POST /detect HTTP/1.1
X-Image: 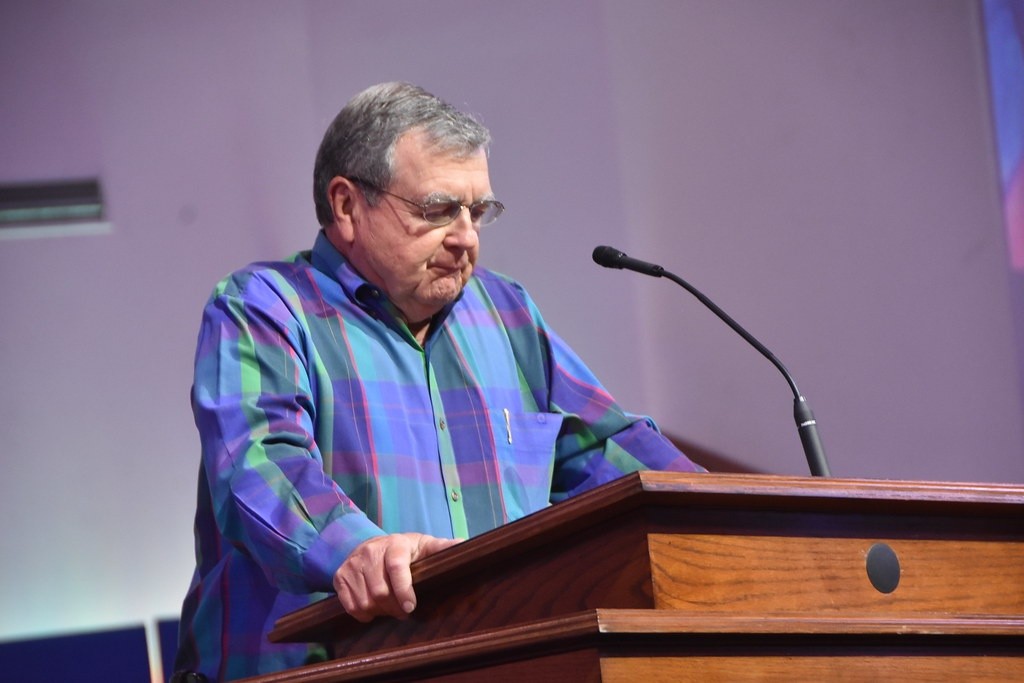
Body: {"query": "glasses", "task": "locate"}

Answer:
[347,176,506,227]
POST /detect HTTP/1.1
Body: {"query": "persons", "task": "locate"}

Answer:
[173,81,708,683]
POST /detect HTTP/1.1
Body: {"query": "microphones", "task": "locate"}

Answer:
[592,245,830,479]
